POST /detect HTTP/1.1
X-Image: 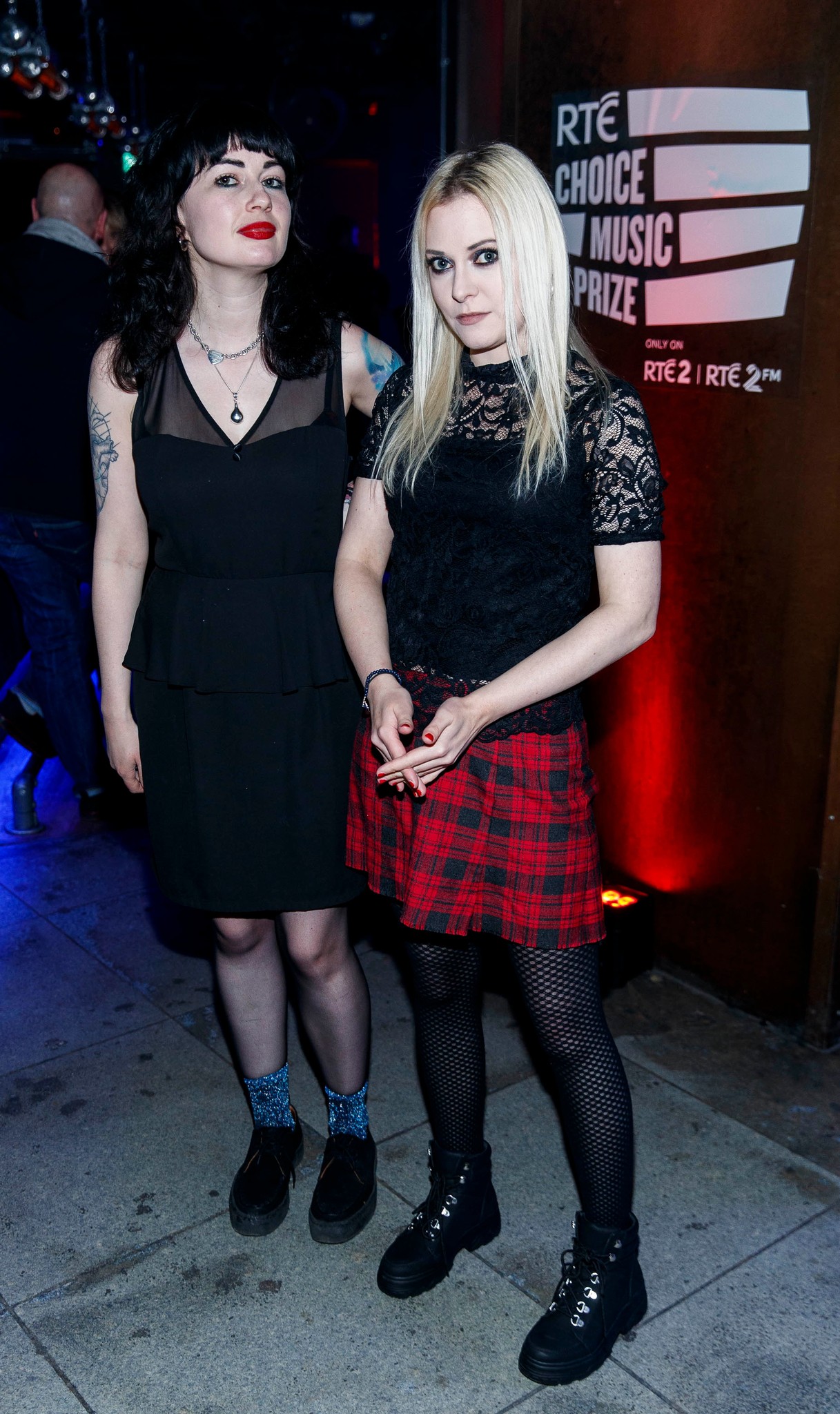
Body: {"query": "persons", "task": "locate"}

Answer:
[89,100,405,1245]
[333,146,663,1385]
[0,156,132,848]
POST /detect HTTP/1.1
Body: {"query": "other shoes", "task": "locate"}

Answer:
[80,788,149,829]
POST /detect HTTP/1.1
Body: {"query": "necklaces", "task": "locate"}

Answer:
[183,315,265,364]
[185,311,264,425]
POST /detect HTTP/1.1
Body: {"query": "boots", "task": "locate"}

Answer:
[518,1210,645,1386]
[379,1142,502,1299]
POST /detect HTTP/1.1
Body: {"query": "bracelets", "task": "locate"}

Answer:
[361,668,402,713]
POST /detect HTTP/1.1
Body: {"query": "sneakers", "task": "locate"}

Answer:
[308,1124,376,1246]
[228,1106,302,1236]
